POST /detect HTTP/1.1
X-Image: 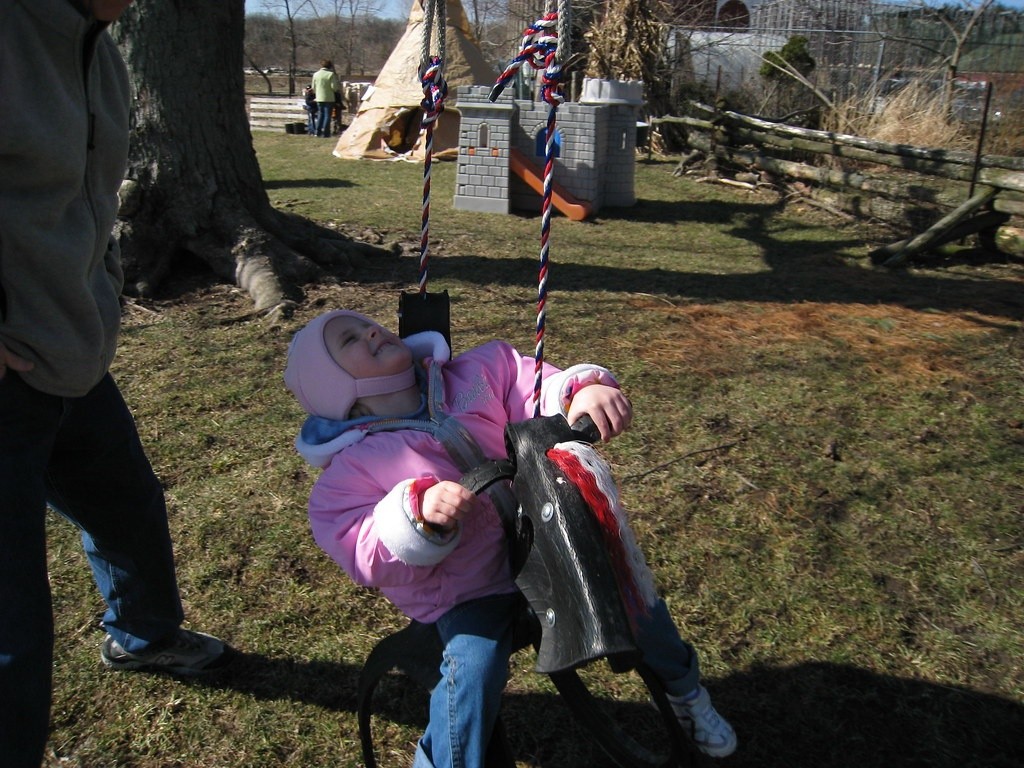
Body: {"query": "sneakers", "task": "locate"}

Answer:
[100,627,235,675]
[649,682,737,758]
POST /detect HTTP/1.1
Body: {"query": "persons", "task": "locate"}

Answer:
[304,61,346,139]
[285,312,738,768]
[0,1,239,768]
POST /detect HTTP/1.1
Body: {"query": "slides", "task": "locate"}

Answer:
[511,148,593,223]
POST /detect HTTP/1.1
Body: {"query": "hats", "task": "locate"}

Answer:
[282,310,415,420]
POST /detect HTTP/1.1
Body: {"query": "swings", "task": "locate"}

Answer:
[355,0,684,768]
